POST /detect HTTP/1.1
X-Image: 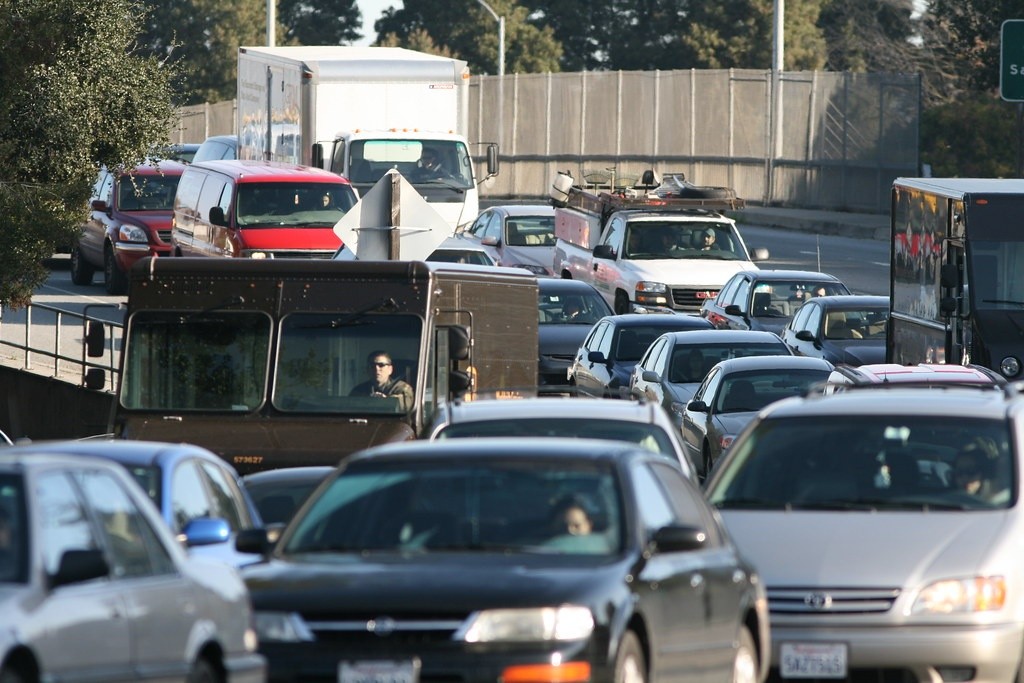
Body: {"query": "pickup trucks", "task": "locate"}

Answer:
[551,206,771,315]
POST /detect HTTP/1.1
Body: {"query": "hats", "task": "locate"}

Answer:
[704,228,716,237]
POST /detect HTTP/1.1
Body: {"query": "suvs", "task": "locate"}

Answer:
[70,157,187,296]
[822,362,1024,500]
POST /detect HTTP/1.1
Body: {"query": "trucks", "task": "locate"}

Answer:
[235,44,499,239]
[886,179,1023,383]
[85,254,539,486]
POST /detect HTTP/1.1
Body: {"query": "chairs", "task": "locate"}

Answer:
[391,360,418,389]
[363,477,463,548]
[447,476,503,548]
[348,158,372,183]
[753,293,784,316]
[562,298,586,319]
[169,351,258,410]
[828,320,854,338]
[724,381,757,409]
[508,222,527,245]
[619,332,639,359]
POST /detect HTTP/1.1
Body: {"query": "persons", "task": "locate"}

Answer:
[549,494,593,538]
[409,147,452,183]
[948,451,991,509]
[678,348,706,381]
[556,296,586,324]
[810,286,826,298]
[350,349,413,425]
[313,193,337,212]
[629,227,721,255]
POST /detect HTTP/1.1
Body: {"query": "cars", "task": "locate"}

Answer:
[429,396,704,496]
[0,437,337,587]
[536,278,622,381]
[700,395,1023,682]
[785,295,890,367]
[151,135,239,167]
[467,205,561,277]
[570,313,719,400]
[629,328,800,430]
[0,456,264,683]
[680,356,836,482]
[239,439,770,683]
[699,269,857,342]
[425,241,497,268]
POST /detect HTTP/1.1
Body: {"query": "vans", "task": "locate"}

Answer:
[172,159,361,262]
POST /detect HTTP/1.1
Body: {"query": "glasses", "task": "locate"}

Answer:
[422,156,433,160]
[369,363,391,367]
